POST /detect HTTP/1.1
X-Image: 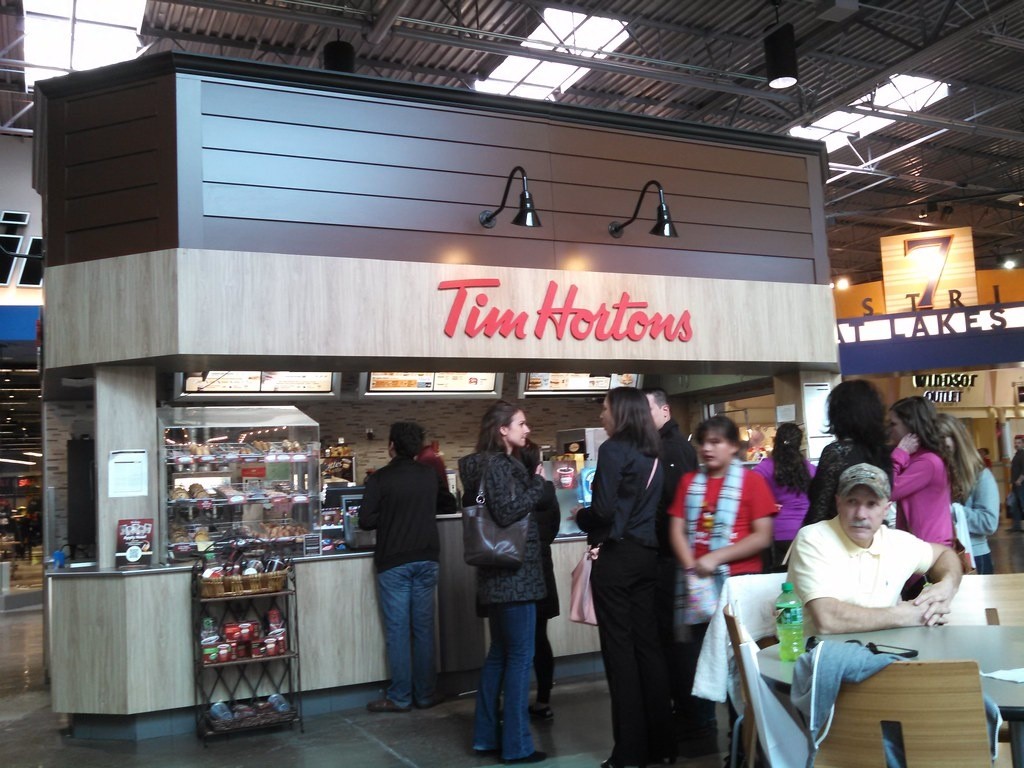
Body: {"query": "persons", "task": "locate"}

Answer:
[459,400,560,764]
[640,387,719,759]
[938,414,1001,574]
[801,380,894,528]
[887,395,953,547]
[358,423,456,712]
[418,437,449,491]
[667,416,779,766]
[567,387,680,767]
[785,463,962,637]
[1006,435,1024,533]
[752,424,816,573]
[978,448,991,469]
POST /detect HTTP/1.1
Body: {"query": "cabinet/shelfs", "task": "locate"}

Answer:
[157,406,322,564]
[190,535,304,748]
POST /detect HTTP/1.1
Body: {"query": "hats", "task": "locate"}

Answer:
[1015,435,1024,439]
[837,463,891,503]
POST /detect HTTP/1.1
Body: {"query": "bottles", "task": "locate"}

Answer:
[774,582,806,662]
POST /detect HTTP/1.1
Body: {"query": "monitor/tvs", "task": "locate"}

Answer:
[341,494,365,543]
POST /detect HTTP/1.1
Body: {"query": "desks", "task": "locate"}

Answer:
[756,625,1024,768]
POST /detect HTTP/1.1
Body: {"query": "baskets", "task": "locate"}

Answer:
[206,705,297,731]
[198,566,292,598]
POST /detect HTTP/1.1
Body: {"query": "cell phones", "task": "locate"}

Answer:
[874,644,919,658]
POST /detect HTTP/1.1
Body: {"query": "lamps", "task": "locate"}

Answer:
[324,29,354,73]
[479,166,542,229]
[763,0,799,89]
[608,180,677,238]
[941,206,953,223]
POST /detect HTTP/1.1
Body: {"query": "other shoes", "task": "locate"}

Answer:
[503,751,546,764]
[413,696,437,709]
[368,699,411,711]
[474,744,502,756]
[528,704,554,721]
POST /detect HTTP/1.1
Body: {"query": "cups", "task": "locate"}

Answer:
[201,557,288,664]
[556,467,575,488]
[210,702,232,720]
[267,693,289,711]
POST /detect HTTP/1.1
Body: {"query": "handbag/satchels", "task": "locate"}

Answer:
[568,548,599,626]
[949,503,977,575]
[463,452,531,571]
[683,563,730,626]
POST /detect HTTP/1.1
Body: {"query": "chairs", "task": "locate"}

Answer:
[691,572,1023,767]
